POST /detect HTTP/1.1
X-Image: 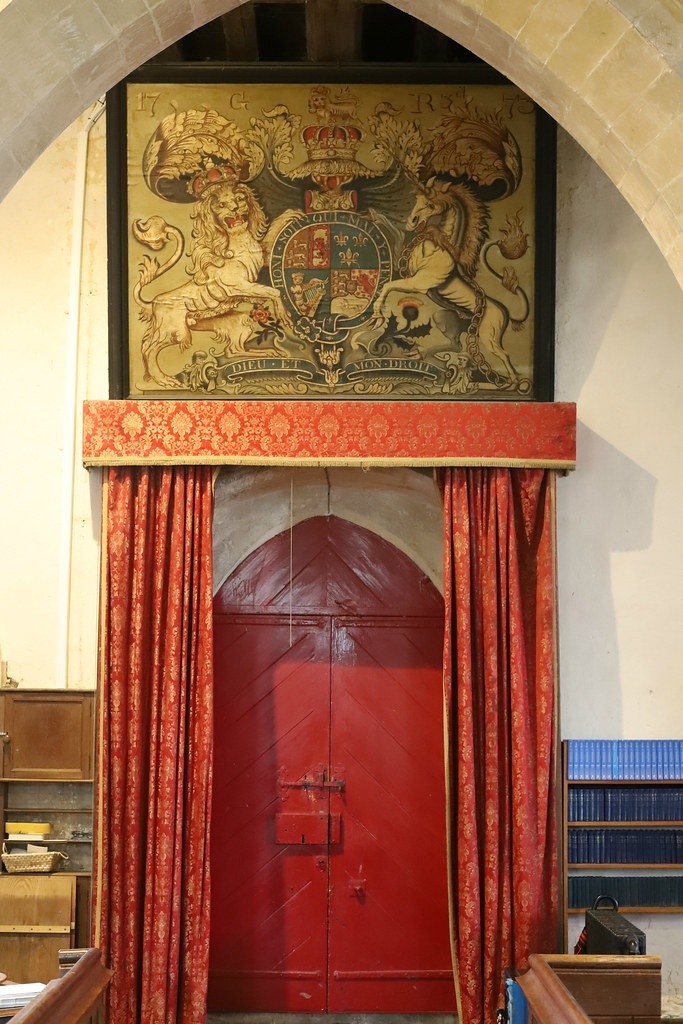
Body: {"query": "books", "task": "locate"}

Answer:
[568,740,683,908]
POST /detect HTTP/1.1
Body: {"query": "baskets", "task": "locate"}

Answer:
[1,843,68,873]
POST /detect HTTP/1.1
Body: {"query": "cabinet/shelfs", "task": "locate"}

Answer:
[0,689,97,947]
[563,739,683,954]
